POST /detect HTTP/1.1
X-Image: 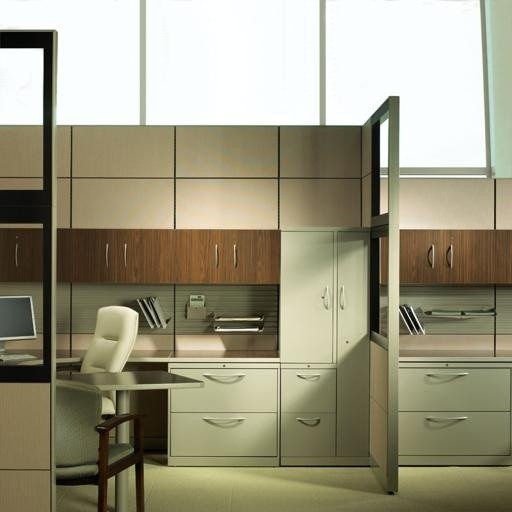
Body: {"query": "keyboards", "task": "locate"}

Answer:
[0,354,39,362]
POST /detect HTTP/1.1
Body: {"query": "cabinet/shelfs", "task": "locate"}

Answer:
[398,357,512,466]
[68,229,173,284]
[173,229,280,285]
[168,362,280,466]
[379,229,512,285]
[280,229,370,467]
[0,227,68,283]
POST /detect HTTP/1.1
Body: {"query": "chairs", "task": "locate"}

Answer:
[80,305,139,416]
[56,378,145,512]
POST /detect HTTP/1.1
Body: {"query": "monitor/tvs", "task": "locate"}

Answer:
[0,296,37,353]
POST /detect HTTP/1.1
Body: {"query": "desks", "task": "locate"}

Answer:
[57,371,204,512]
